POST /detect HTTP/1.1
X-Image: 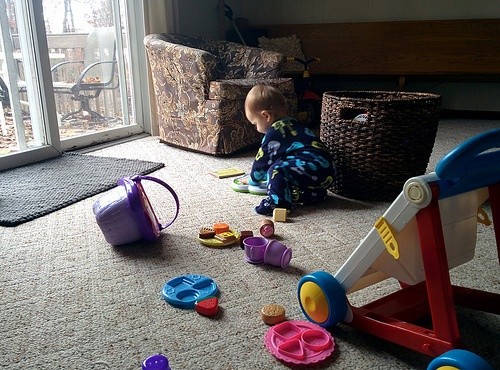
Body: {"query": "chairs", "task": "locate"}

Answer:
[50,29,123,124]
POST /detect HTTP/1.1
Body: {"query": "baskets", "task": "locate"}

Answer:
[319,88,442,201]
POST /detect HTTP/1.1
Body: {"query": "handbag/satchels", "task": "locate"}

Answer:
[93,174,180,246]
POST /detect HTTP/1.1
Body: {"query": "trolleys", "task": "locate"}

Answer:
[297,128,500,370]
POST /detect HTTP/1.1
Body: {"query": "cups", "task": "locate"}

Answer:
[242,236,268,264]
[263,239,292,269]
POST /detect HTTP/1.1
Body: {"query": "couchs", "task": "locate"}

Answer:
[143,33,295,156]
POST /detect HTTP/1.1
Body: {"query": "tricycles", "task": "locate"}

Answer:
[222,2,323,130]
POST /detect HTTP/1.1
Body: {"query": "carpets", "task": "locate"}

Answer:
[0,153,165,226]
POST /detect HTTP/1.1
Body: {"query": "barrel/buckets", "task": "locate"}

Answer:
[92,175,179,248]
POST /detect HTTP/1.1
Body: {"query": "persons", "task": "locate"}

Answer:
[241,84,337,215]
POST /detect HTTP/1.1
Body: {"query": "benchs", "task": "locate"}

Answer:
[242,18,499,92]
